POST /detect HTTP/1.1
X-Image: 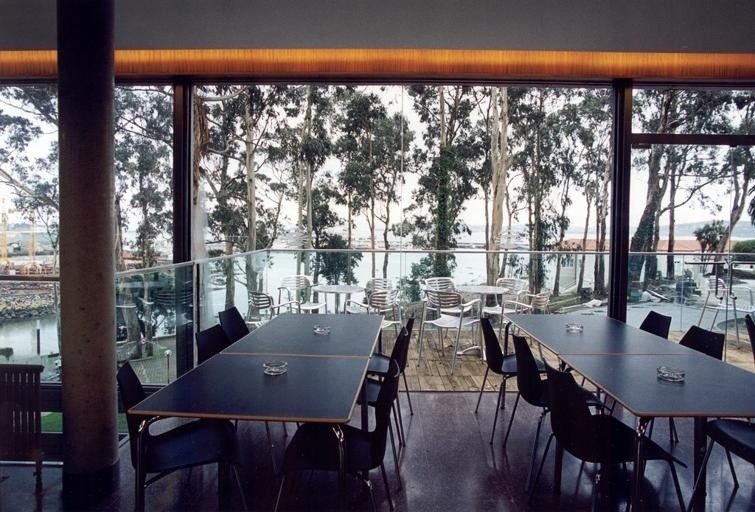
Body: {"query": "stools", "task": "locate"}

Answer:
[686,419,755,512]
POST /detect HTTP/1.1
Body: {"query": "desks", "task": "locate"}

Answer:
[129,353,371,512]
[219,313,387,482]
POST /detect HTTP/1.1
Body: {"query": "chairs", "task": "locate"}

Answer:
[273,358,401,512]
[745,315,755,358]
[116,361,249,511]
[356,326,410,490]
[276,275,327,314]
[367,312,417,416]
[0,364,44,495]
[219,307,250,343]
[697,277,755,350]
[195,324,288,447]
[362,278,399,318]
[246,292,301,323]
[646,326,725,449]
[526,359,686,512]
[602,311,672,416]
[344,290,399,338]
